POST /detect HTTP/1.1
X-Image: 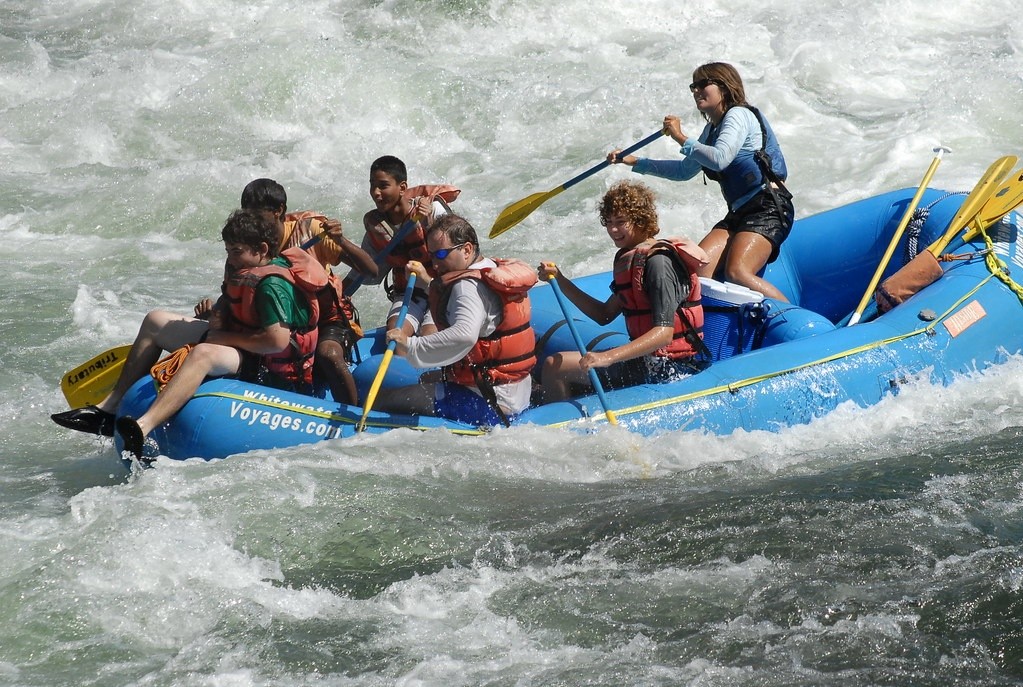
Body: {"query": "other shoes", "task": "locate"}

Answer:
[116,415,144,462]
[51,404,117,438]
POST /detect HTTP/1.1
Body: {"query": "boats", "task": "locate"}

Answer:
[113,187,1023,461]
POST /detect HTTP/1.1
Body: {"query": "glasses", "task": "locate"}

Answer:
[427,244,465,260]
[689,78,725,93]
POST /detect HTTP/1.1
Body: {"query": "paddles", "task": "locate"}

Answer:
[340,212,424,299]
[59,228,334,413]
[545,262,620,427]
[488,125,672,240]
[354,259,419,435]
[834,143,1023,329]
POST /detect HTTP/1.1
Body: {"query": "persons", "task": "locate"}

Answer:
[536,178,711,405]
[372,214,540,425]
[194,178,379,406]
[50,208,328,461]
[341,155,462,357]
[606,62,795,304]
